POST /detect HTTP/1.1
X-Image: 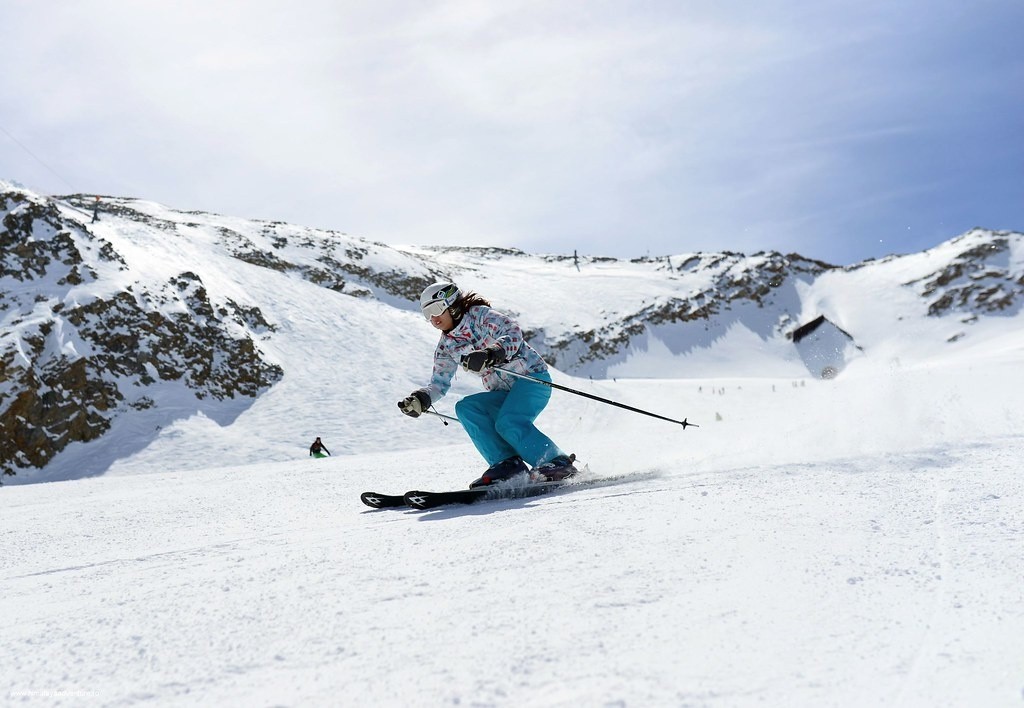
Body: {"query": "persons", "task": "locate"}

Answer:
[309,437,331,458]
[397,282,581,491]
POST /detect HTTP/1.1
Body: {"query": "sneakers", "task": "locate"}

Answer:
[529,455,578,483]
[469,456,529,490]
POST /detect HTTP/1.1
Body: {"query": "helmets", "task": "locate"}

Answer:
[420,282,462,322]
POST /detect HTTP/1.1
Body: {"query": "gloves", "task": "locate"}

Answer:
[462,344,506,375]
[397,390,432,417]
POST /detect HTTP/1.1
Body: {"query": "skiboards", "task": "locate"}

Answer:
[360,466,667,510]
